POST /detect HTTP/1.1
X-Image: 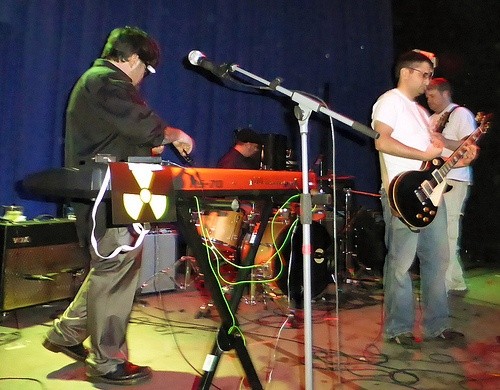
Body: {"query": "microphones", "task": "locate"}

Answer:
[188,50,232,81]
[23,273,54,281]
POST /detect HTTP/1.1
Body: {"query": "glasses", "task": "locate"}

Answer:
[138,56,156,77]
[406,66,433,80]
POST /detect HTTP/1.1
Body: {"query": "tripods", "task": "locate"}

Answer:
[328,186,381,287]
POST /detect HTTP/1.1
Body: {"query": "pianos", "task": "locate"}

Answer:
[164,161,318,193]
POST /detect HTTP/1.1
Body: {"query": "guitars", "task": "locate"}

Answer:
[388,111,494,231]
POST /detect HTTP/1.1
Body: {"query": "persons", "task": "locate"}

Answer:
[205,128,264,226]
[371,50,477,349]
[40,26,193,386]
[417,78,478,301]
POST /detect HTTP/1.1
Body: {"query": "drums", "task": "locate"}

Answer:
[254,217,336,308]
[193,203,251,253]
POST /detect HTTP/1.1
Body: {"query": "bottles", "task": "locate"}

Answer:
[260,144,267,170]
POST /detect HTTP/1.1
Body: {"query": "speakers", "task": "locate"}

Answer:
[0,218,91,312]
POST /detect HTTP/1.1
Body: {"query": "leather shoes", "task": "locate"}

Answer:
[86,359,153,386]
[41,334,89,362]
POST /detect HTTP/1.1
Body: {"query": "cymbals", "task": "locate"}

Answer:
[317,170,356,184]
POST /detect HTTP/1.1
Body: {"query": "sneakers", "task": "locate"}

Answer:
[424,329,465,344]
[384,332,425,349]
